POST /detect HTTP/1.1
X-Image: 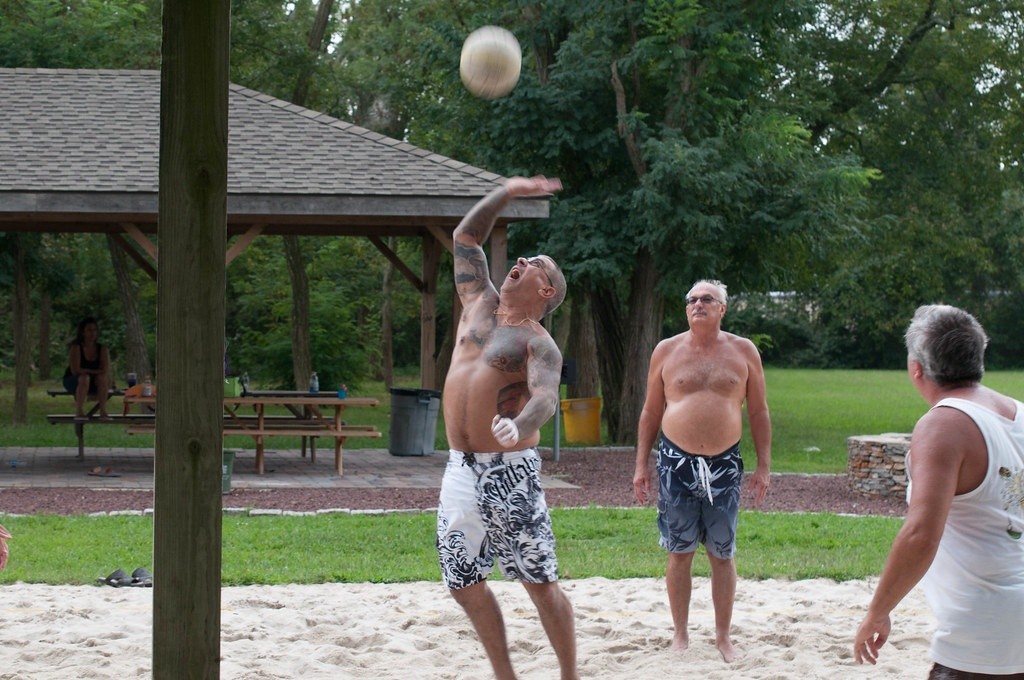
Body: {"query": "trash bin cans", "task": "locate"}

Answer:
[223,376,242,398]
[389,386,442,456]
[560,397,601,447]
[221,450,237,494]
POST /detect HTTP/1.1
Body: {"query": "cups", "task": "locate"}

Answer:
[338,387,346,398]
[129,373,136,387]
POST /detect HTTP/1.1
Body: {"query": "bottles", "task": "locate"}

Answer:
[309,372,319,392]
[142,376,151,397]
[243,372,249,390]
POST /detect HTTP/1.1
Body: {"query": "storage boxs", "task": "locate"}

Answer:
[223,375,242,398]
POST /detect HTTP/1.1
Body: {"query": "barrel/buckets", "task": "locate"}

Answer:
[559,396,601,445]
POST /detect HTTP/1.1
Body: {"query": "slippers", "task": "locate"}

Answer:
[132,568,152,587]
[98,568,145,587]
[98,467,121,476]
[88,466,101,475]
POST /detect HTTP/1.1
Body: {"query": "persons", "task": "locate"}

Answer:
[852,302,1024,680]
[435,176,582,680]
[633,281,773,662]
[62,316,114,423]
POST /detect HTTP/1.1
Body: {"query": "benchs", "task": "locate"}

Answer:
[46,414,383,448]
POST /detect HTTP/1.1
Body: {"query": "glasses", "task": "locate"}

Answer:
[686,295,722,305]
[528,259,553,287]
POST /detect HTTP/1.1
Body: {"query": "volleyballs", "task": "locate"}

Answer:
[459,25,523,102]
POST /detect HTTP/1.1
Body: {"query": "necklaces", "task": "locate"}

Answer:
[494,307,542,326]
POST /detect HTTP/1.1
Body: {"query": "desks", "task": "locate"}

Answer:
[47,387,127,461]
[242,390,345,463]
[125,395,378,474]
